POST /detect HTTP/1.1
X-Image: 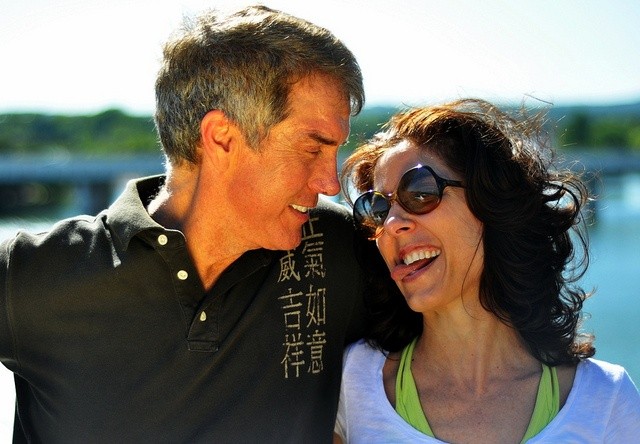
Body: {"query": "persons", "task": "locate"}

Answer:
[0,6,423,444]
[333,98,640,444]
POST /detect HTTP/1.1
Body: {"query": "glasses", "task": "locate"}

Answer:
[353,165,467,230]
